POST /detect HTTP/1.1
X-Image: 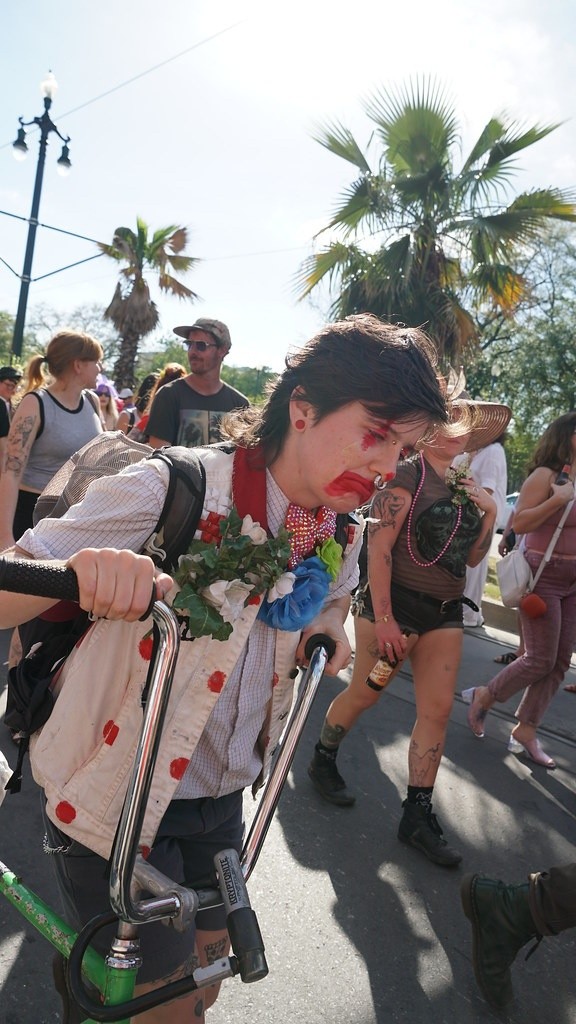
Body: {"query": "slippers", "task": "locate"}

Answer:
[494,651,518,664]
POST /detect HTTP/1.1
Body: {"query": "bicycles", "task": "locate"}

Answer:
[0,554,339,1024]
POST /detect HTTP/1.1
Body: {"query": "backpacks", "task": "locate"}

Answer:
[7,430,347,735]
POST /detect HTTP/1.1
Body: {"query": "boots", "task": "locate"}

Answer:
[308,743,356,805]
[463,869,542,1011]
[398,798,462,865]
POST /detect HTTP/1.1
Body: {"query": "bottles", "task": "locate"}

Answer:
[364,627,412,691]
[549,463,571,498]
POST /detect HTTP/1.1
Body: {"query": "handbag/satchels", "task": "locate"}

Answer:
[496,552,535,606]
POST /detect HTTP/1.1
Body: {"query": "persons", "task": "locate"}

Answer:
[0,313,479,1024]
[0,331,105,672]
[450,451,479,471]
[461,413,576,769]
[307,390,512,866]
[0,364,24,439]
[142,317,251,449]
[125,363,188,443]
[564,683,576,693]
[493,467,527,663]
[460,862,576,1024]
[113,371,165,436]
[118,388,135,408]
[92,381,119,431]
[462,429,511,627]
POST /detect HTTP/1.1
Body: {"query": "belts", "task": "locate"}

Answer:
[389,581,481,614]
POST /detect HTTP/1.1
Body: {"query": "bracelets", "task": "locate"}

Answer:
[375,614,393,622]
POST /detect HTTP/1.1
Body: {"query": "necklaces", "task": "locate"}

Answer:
[406,451,462,567]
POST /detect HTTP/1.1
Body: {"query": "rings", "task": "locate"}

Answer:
[385,643,391,649]
[473,485,478,492]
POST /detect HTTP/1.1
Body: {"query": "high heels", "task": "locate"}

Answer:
[507,734,556,767]
[463,688,488,737]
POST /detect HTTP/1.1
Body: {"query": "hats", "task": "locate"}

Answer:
[173,318,232,351]
[441,385,514,453]
[0,367,26,379]
[119,387,134,399]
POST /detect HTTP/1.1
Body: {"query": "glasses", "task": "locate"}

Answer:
[182,340,218,352]
[95,392,111,398]
[3,381,18,389]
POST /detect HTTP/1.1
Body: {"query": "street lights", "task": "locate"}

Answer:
[6,66,74,368]
